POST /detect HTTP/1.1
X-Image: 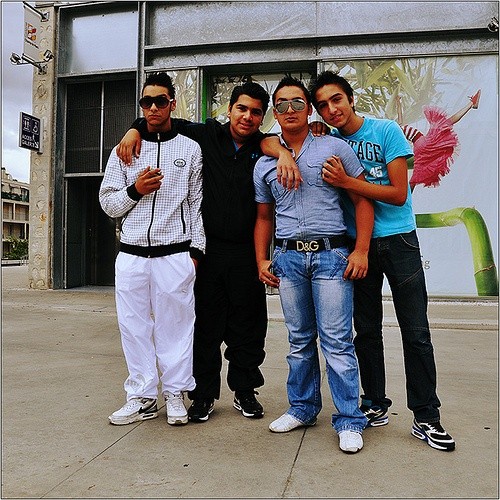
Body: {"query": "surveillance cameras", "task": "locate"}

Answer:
[9,51,21,64]
[42,49,55,61]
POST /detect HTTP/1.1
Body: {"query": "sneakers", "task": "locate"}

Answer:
[233,394,265,419]
[360,395,392,430]
[164,394,188,425]
[187,400,214,423]
[338,431,363,454]
[269,413,317,433]
[411,419,456,452]
[108,399,158,426]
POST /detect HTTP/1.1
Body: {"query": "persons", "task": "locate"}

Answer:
[115,81,331,421]
[401,89,481,186]
[98,72,206,424]
[261,72,455,451]
[253,77,374,453]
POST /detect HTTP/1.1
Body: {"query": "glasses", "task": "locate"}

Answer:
[274,99,310,115]
[139,96,174,109]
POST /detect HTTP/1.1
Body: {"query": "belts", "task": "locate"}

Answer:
[276,232,349,252]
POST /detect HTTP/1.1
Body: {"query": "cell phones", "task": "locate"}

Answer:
[147,169,161,184]
